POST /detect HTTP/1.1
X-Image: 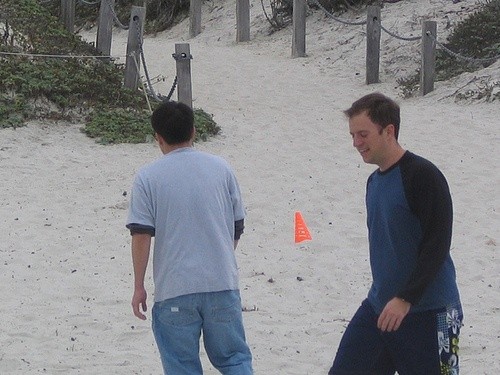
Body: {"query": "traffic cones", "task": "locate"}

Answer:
[294,211,312,243]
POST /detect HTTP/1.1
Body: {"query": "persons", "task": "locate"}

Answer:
[328,93,464,375]
[126,102,254,375]
